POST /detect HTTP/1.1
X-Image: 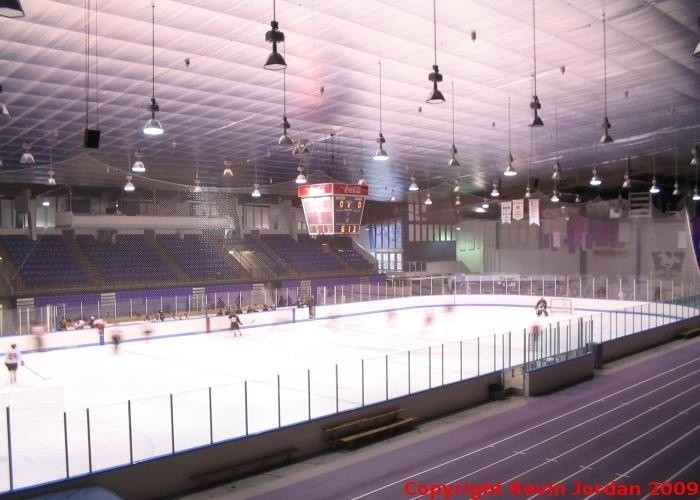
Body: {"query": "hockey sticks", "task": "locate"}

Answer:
[23,364,52,381]
[242,320,255,324]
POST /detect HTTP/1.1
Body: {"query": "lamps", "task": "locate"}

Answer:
[0,0,700,213]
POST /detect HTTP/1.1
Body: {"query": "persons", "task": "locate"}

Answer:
[4,293,315,385]
[438,270,667,316]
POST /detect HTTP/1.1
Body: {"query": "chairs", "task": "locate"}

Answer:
[1,230,390,324]
